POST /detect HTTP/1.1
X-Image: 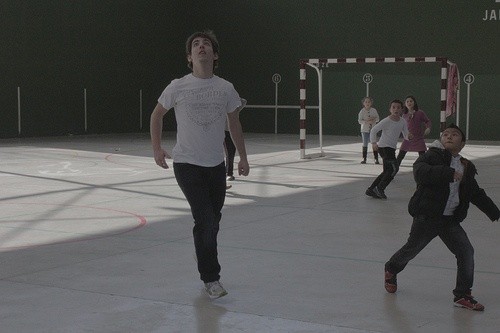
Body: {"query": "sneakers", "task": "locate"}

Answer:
[384,265,397,293]
[204,281,227,298]
[366,188,380,198]
[372,186,387,199]
[453,296,485,311]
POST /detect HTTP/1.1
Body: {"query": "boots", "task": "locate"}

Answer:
[360,147,367,164]
[373,150,379,164]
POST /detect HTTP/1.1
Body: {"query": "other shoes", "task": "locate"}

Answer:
[226,176,234,180]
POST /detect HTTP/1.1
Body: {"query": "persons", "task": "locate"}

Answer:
[150,28,250,298]
[384,123,500,312]
[223,97,247,190]
[395,96,432,166]
[358,97,381,164]
[365,99,416,199]
